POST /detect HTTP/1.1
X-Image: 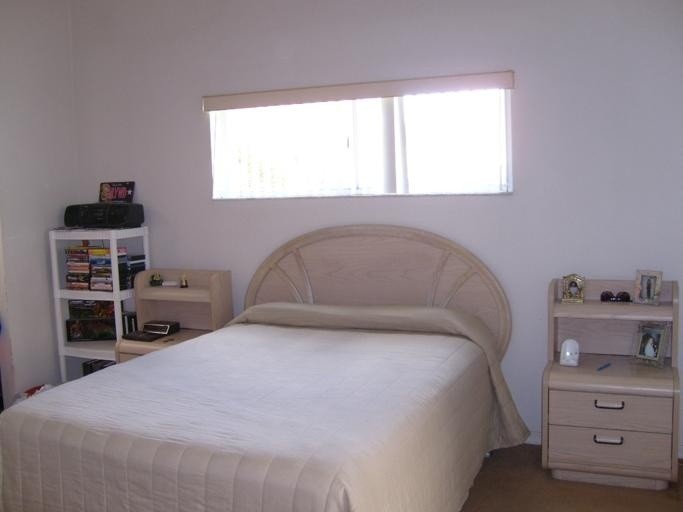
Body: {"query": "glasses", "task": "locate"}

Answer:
[601,291,633,302]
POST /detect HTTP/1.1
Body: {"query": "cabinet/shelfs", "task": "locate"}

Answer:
[49,227,150,381]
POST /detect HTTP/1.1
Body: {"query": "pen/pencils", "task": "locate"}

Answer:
[597,362,611,370]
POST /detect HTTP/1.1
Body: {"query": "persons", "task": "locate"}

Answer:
[644,337,658,358]
[568,281,578,295]
[645,278,652,300]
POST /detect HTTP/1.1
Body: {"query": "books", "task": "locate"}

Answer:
[130,316,136,332]
[122,312,131,334]
[82,359,116,377]
[63,244,146,293]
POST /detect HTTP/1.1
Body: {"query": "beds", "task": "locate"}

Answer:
[1,227,533,512]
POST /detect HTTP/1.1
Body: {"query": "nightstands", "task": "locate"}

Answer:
[116,265,235,361]
[540,282,683,492]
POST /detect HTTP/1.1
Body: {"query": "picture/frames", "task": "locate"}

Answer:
[632,269,664,307]
[630,322,672,369]
[561,274,586,305]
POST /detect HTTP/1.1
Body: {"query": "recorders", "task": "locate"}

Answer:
[64,203,144,227]
